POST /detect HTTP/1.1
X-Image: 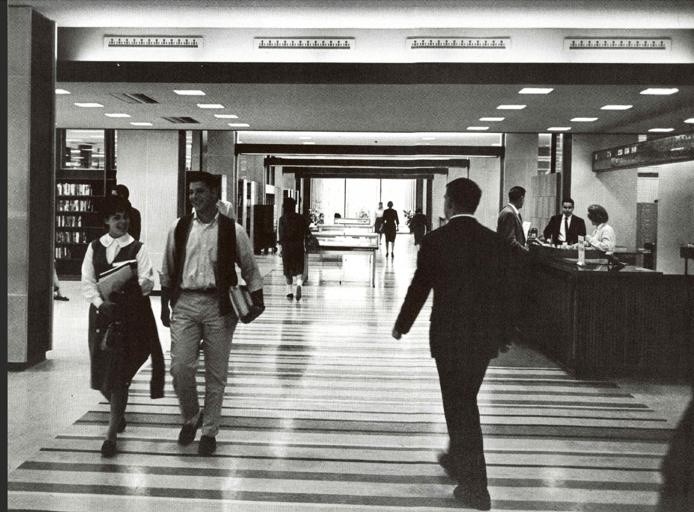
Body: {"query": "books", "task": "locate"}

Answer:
[55,182,94,259]
[226,282,253,318]
[98,259,138,301]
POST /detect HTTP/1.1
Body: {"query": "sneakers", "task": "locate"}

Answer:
[296,285,301,301]
[287,294,293,300]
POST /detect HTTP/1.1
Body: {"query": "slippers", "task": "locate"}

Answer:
[54,293,69,301]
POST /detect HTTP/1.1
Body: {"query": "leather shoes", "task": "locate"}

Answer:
[199,433,218,456]
[101,428,117,458]
[437,453,462,482]
[178,410,203,446]
[117,418,126,433]
[453,482,491,510]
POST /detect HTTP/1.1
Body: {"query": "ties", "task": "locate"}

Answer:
[563,216,572,240]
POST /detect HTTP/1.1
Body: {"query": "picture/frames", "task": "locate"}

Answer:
[59,128,105,172]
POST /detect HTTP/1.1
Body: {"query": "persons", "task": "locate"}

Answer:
[391,177,529,509]
[543,197,586,244]
[190,176,237,222]
[304,207,325,226]
[277,197,305,302]
[104,184,141,242]
[83,195,155,459]
[53,261,71,301]
[408,208,432,248]
[156,170,265,456]
[372,202,385,245]
[379,201,401,258]
[585,205,616,257]
[497,185,529,354]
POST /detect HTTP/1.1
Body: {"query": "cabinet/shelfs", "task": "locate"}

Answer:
[56,168,115,281]
[247,181,259,255]
[264,183,277,232]
[237,177,248,234]
[215,173,227,200]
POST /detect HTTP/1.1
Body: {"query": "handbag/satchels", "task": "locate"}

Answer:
[304,231,320,253]
